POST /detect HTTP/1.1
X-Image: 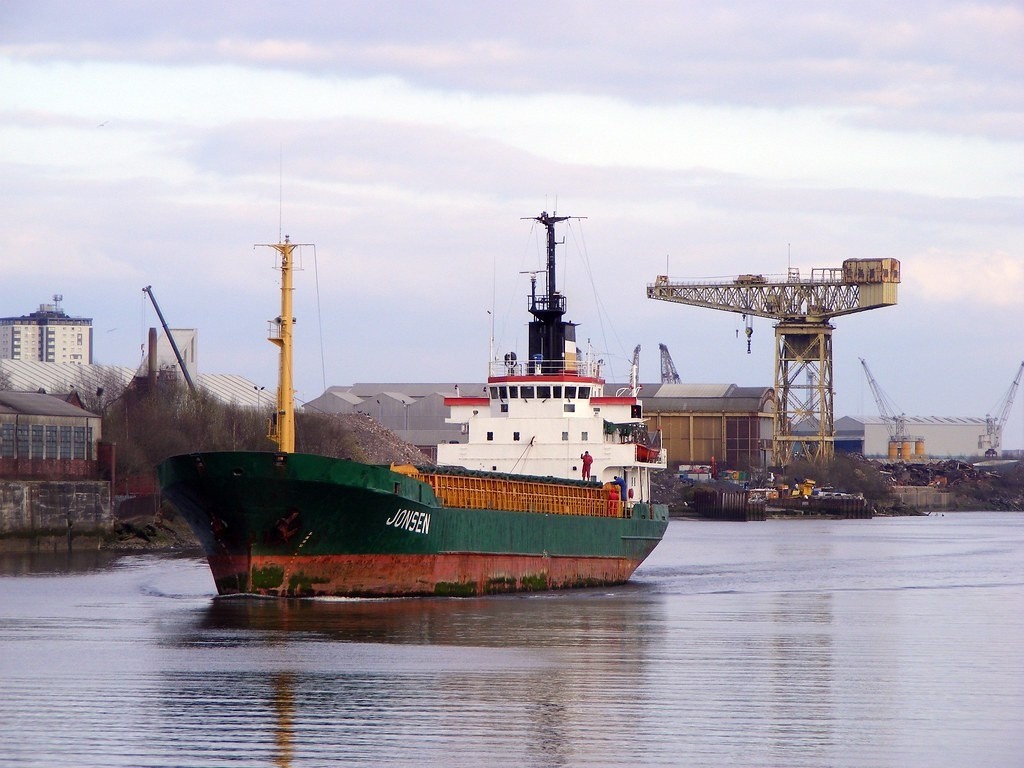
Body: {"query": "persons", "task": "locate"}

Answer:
[581,451,593,481]
[610,476,627,501]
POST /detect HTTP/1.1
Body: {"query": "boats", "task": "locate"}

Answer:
[155,212,670,597]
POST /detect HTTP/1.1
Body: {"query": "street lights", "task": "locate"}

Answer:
[253,386,265,412]
